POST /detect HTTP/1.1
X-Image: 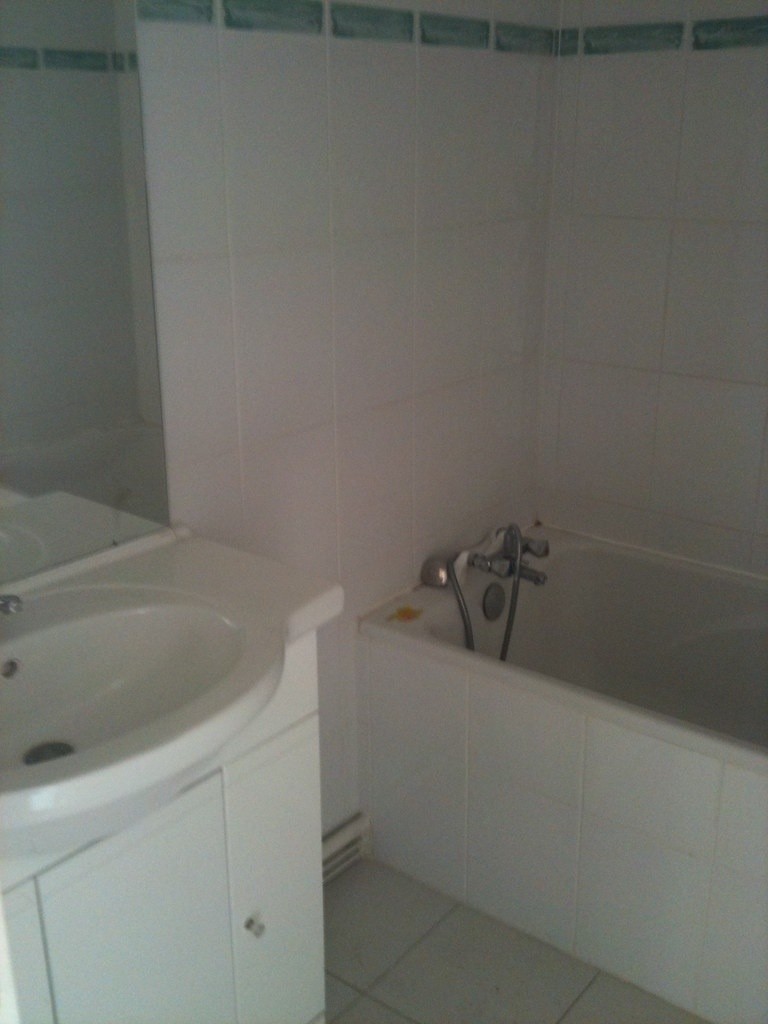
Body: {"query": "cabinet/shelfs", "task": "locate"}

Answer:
[1,766,236,1024]
[221,710,328,1024]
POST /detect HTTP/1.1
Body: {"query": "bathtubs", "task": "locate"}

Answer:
[360,520,768,1024]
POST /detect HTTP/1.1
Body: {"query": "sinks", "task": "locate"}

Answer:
[0,584,285,826]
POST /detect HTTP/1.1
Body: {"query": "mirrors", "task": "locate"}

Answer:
[0,0,188,608]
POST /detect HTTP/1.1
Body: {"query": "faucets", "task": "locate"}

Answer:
[0,594,24,615]
[503,558,548,587]
